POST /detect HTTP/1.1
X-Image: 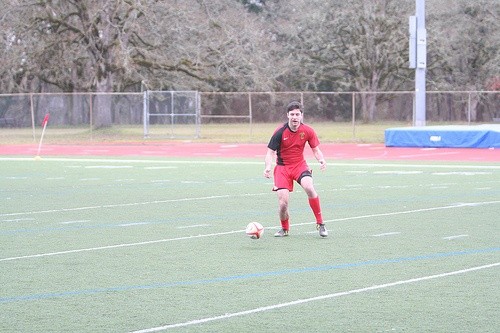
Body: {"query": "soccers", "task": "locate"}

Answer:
[244,222,265,240]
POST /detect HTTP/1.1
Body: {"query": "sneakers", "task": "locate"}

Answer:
[316,223,328,237]
[273,229,290,237]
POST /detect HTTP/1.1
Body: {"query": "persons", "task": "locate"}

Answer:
[263,101,328,237]
